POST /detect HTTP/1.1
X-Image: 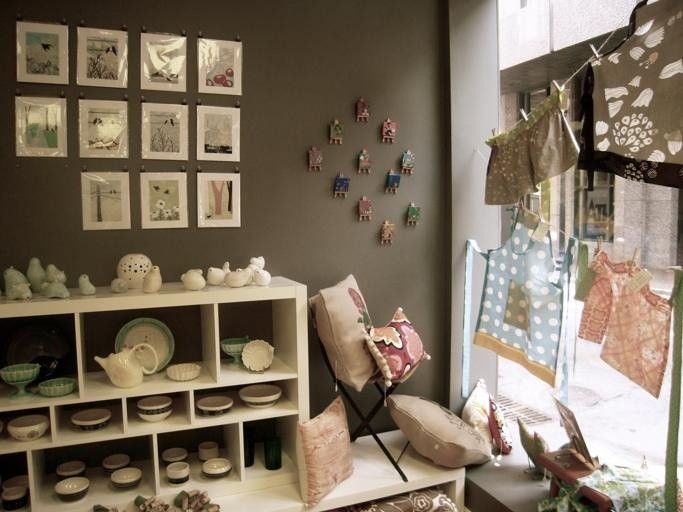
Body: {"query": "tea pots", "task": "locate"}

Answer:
[92,343,156,387]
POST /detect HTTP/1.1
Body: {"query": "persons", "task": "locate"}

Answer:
[384,123,393,144]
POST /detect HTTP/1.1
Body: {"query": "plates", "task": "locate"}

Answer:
[112,317,176,375]
[241,338,274,372]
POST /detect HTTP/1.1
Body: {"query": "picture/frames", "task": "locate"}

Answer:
[198,38,243,96]
[197,105,240,162]
[142,103,189,161]
[197,172,240,228]
[79,99,129,159]
[76,28,128,89]
[81,172,131,230]
[141,33,187,93]
[15,96,67,158]
[140,172,188,230]
[16,21,70,85]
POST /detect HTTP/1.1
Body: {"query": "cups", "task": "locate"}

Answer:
[160,446,190,488]
[198,440,222,460]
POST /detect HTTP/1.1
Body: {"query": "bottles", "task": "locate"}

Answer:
[243,426,254,467]
[263,426,282,470]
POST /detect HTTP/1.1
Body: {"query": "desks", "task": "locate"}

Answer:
[534,451,668,512]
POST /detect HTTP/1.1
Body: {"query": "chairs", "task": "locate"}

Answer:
[306,293,419,482]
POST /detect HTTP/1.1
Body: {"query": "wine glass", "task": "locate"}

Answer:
[222,336,248,365]
[0,361,42,400]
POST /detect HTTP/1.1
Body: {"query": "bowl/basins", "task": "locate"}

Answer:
[134,394,174,423]
[201,458,231,477]
[6,414,49,442]
[193,395,234,415]
[0,470,31,509]
[237,383,282,408]
[70,407,111,432]
[51,450,142,503]
[39,376,76,398]
[167,362,202,382]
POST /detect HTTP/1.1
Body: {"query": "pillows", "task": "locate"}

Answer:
[486,396,512,453]
[356,303,429,382]
[315,272,380,392]
[385,395,493,468]
[516,420,553,479]
[294,392,353,512]
[458,377,493,453]
[334,487,458,512]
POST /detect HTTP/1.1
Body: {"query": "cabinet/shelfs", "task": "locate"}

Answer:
[0,274,310,512]
[163,426,469,512]
